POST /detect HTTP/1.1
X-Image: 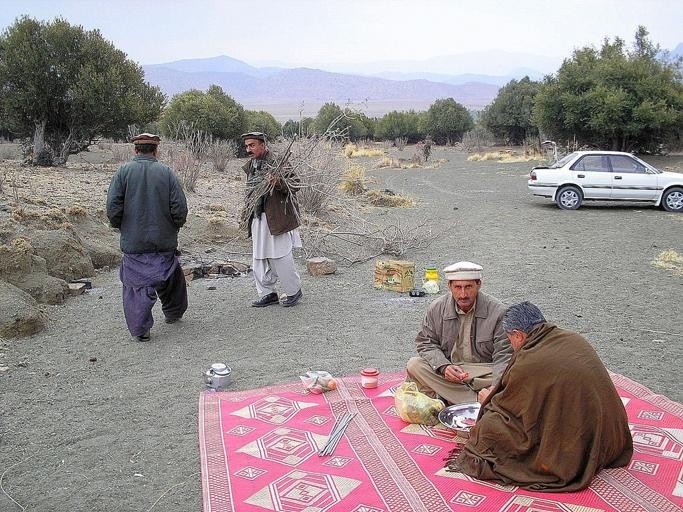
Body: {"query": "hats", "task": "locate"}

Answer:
[442,261,483,281]
[129,133,161,144]
[241,132,268,140]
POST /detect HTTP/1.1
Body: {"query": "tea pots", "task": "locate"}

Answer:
[203,362,232,390]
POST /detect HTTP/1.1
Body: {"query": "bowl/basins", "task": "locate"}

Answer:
[438,400,481,438]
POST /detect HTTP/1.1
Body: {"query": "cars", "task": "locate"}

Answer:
[526,150,682,213]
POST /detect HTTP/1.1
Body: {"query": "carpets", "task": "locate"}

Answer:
[198,366,682,512]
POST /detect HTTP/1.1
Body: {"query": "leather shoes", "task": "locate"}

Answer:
[252,292,280,306]
[282,290,303,307]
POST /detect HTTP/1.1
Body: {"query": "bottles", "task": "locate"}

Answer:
[360,368,380,389]
[424,267,439,283]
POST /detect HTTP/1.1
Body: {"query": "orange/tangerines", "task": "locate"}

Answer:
[327,380,339,390]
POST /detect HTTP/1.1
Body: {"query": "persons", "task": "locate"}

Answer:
[105,131,190,343]
[240,128,304,308]
[405,261,512,407]
[442,300,634,494]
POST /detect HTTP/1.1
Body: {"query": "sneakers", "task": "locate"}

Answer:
[137,336,150,342]
